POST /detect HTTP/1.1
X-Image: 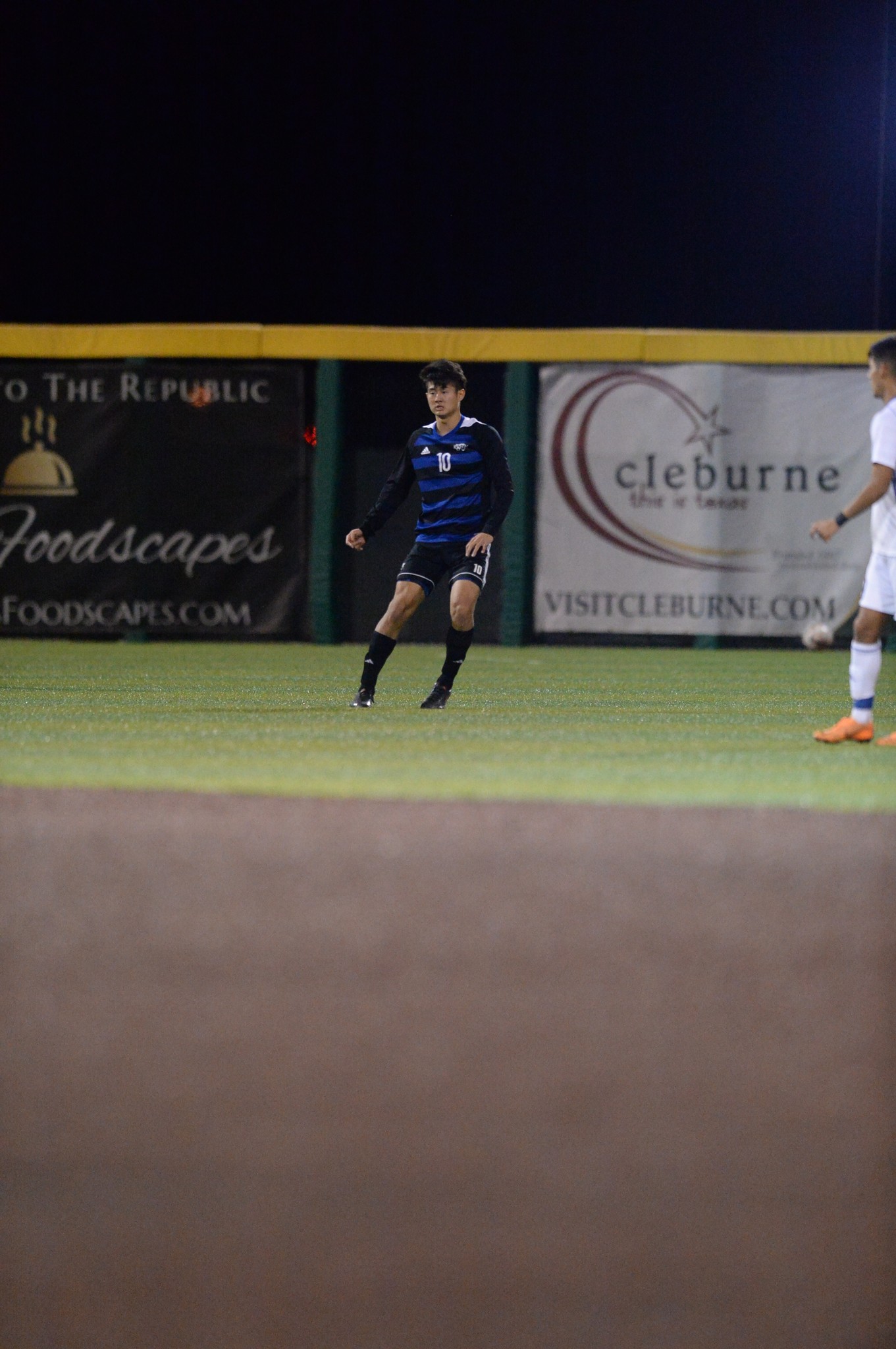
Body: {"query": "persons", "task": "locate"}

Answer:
[810,336,896,747]
[344,356,515,709]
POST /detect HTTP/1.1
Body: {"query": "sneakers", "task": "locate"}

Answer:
[350,686,375,707]
[814,717,873,743]
[421,683,452,710]
[878,731,896,746]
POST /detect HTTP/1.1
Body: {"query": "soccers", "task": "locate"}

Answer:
[800,620,835,652]
